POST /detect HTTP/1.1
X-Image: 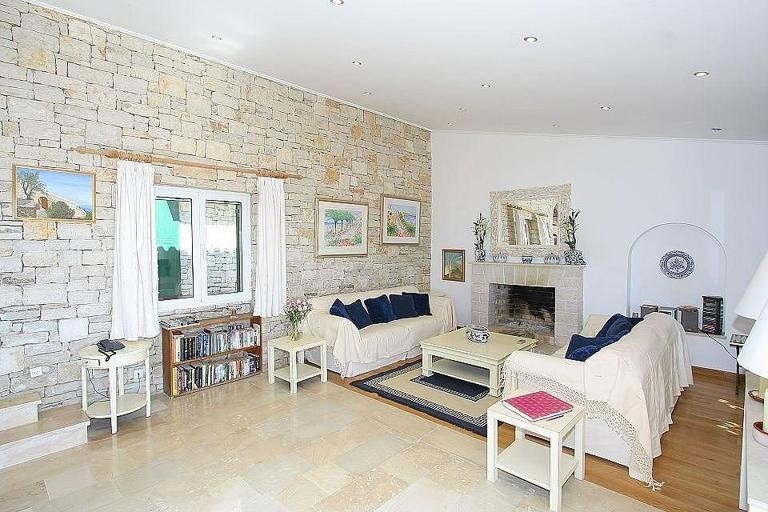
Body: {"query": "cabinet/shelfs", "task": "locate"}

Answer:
[160,311,264,401]
[738,370,768,512]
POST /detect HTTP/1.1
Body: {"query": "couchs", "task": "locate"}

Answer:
[299,282,459,385]
[497,309,691,472]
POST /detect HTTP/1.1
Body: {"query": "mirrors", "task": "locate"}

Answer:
[487,184,574,261]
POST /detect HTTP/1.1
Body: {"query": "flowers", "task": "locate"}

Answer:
[282,298,316,337]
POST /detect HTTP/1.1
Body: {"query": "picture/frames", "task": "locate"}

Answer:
[312,195,371,259]
[378,193,424,248]
[439,246,467,283]
[10,161,99,228]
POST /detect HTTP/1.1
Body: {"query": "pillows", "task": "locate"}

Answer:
[344,299,373,332]
[363,293,398,326]
[411,294,434,317]
[388,293,419,320]
[328,297,349,319]
[564,312,645,362]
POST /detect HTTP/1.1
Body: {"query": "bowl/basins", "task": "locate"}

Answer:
[544,254,561,265]
[465,324,491,344]
[522,255,533,263]
[494,255,507,262]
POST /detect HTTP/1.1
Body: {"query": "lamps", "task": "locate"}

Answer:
[735,253,768,405]
[736,299,768,447]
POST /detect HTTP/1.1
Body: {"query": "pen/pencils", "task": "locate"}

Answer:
[546,415,565,422]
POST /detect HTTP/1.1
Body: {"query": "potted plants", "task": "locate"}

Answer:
[468,212,491,263]
[556,208,584,267]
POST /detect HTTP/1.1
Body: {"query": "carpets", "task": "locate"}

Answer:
[349,355,505,438]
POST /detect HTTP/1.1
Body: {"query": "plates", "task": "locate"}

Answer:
[659,250,695,279]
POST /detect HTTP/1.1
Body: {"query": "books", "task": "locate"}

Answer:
[173,317,261,397]
[501,389,575,422]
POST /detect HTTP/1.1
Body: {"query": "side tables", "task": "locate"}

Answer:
[267,333,329,392]
[485,387,588,512]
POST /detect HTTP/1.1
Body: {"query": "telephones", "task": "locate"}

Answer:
[97,339,125,352]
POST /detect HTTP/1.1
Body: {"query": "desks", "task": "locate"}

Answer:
[75,335,157,437]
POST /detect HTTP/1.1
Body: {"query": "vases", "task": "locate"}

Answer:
[287,319,300,339]
